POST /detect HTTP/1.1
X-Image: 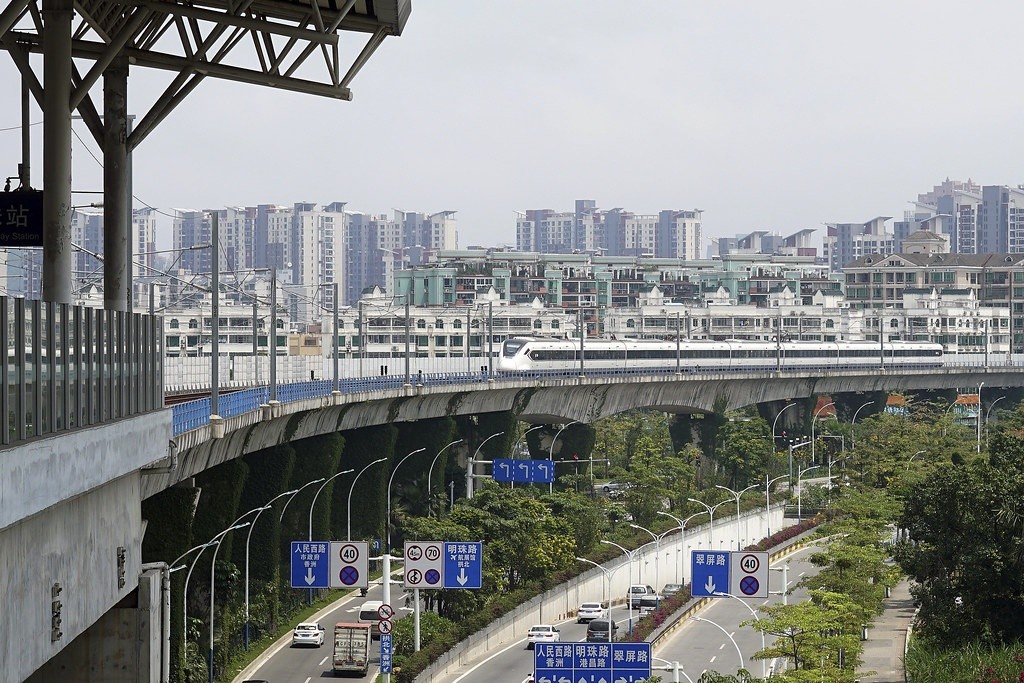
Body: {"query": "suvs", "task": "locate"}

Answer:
[626,583,655,610]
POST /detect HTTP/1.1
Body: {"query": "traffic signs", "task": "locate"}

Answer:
[444,542,482,589]
[534,642,652,683]
[291,541,329,589]
[690,550,730,598]
[380,634,393,674]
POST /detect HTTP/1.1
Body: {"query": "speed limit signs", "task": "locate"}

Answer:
[740,554,760,573]
[425,546,440,561]
[340,544,359,563]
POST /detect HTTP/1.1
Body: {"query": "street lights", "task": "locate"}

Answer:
[713,591,767,683]
[348,417,580,554]
[772,402,873,519]
[944,382,1005,452]
[690,616,744,683]
[575,557,637,642]
[171,469,354,683]
[767,474,790,552]
[601,484,763,638]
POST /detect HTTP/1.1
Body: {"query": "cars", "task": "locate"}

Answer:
[603,478,645,502]
[292,623,326,648]
[662,583,682,600]
[639,596,665,621]
[577,602,619,643]
[527,625,560,650]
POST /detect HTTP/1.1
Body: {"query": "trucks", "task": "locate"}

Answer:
[333,623,373,677]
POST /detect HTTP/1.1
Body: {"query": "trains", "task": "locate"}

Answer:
[496,338,942,375]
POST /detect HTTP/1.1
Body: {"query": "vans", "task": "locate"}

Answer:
[358,601,383,638]
